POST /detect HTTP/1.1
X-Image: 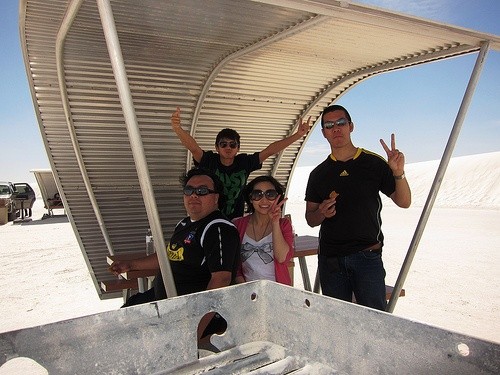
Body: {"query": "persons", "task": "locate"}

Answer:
[24,187,32,217]
[171,106,311,222]
[230,176,295,286]
[109,169,240,309]
[53,191,63,206]
[304,105,410,312]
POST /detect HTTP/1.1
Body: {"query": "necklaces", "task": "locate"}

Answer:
[252,217,270,241]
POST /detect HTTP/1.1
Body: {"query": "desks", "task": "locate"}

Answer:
[106,235,321,294]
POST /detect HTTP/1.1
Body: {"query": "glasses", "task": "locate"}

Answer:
[323,117,350,129]
[183,185,216,196]
[220,141,239,148]
[248,189,278,202]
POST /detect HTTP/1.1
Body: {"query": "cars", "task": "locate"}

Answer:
[0,180,36,220]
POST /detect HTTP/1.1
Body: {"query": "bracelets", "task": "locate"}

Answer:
[394,174,405,180]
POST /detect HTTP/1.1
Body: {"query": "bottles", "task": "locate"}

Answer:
[146,229,155,256]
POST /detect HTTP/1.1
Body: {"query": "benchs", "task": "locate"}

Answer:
[352,283,407,304]
[101,261,295,304]
[44,199,66,216]
